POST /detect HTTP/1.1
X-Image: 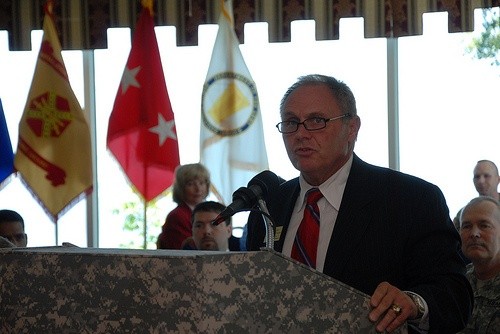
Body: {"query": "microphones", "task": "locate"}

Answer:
[212,170,281,226]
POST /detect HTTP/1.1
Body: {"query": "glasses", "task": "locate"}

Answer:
[276,114,350,133]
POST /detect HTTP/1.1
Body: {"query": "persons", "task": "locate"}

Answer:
[157,163,210,251]
[0,209,27,248]
[452,160,500,266]
[191,201,232,252]
[459,196,500,334]
[246,74,474,334]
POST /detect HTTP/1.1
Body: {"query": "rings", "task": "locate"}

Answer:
[390,303,402,315]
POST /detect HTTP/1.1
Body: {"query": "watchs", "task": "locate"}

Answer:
[405,291,425,320]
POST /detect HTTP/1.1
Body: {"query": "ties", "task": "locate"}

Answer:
[291,188,323,269]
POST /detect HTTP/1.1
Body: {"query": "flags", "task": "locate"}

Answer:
[106,0,180,208]
[199,0,269,207]
[0,98,16,190]
[13,0,93,222]
